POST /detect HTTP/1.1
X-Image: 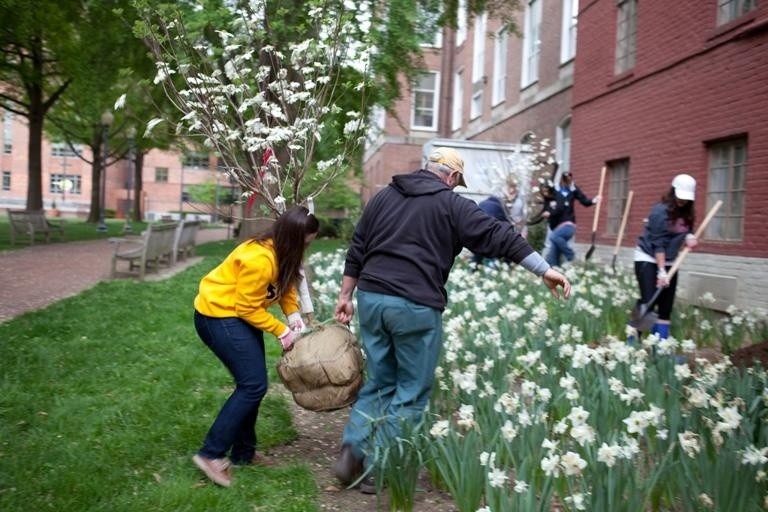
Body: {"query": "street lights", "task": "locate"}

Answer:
[214,171,221,223]
[93,107,115,233]
[179,165,200,220]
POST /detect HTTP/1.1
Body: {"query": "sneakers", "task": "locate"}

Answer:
[332,445,359,485]
[192,451,234,488]
[359,465,395,493]
[237,449,266,465]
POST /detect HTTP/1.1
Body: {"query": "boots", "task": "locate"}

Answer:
[624,313,645,348]
[652,317,686,366]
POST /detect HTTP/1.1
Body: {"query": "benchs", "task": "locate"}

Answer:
[108,220,200,281]
[7,207,65,246]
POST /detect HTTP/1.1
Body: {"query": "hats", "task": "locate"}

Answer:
[426,146,469,191]
[561,171,573,181]
[670,171,698,203]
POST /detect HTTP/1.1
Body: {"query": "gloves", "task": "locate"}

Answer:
[653,267,670,287]
[277,325,297,350]
[287,313,307,332]
[685,234,699,249]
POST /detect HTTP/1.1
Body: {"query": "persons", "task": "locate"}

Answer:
[469,160,602,278]
[193,204,319,487]
[625,172,699,364]
[331,146,573,497]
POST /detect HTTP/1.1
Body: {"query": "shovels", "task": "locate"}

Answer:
[610,190,635,274]
[628,199,725,333]
[585,167,607,260]
